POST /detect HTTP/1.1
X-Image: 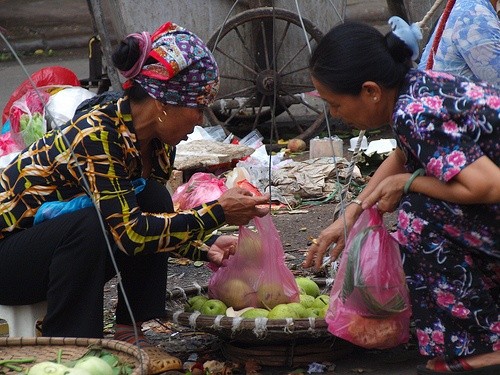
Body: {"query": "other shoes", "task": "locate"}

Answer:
[139,346,183,374]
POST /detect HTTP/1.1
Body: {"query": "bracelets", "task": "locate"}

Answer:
[351,198,364,208]
[405,168,425,193]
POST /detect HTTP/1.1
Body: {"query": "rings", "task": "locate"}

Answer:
[376,202,381,212]
[312,239,319,245]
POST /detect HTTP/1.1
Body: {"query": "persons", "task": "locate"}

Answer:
[304,16,500,375]
[418,0,500,86]
[0,22,270,375]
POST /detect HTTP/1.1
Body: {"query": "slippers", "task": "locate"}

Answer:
[418,353,499,375]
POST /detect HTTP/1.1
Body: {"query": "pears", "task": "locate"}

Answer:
[183,276,331,319]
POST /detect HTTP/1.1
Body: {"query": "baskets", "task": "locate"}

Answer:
[164,277,364,368]
[0,336,150,375]
